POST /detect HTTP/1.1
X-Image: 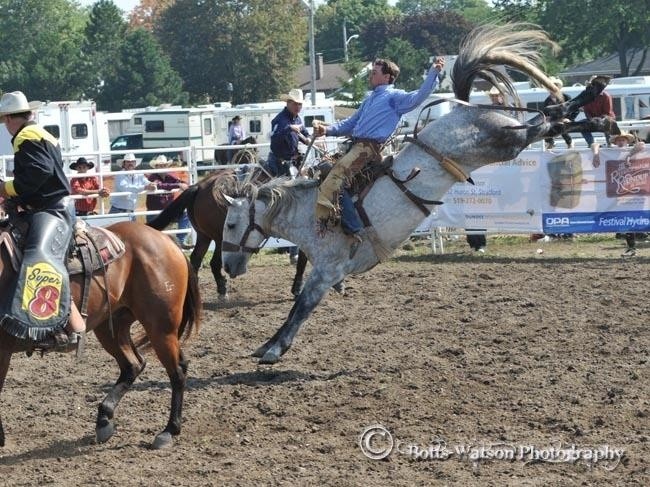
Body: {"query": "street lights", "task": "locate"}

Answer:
[342,20,360,63]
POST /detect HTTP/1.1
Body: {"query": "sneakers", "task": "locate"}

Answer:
[621,247,635,256]
[38,333,78,347]
[348,230,362,259]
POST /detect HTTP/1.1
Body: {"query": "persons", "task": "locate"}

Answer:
[486,85,515,115]
[543,76,579,149]
[267,87,311,178]
[69,157,110,216]
[108,152,157,221]
[590,131,646,257]
[581,77,616,148]
[168,158,195,249]
[146,154,189,223]
[0,91,77,349]
[226,115,245,164]
[312,57,445,260]
[466,230,487,252]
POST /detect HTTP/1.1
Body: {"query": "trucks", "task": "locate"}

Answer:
[111,133,183,170]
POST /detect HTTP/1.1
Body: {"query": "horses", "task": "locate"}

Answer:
[142,149,347,303]
[0,217,203,451]
[209,14,620,366]
[212,134,260,167]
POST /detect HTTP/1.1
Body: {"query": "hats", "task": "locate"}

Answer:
[116,153,142,168]
[280,89,306,104]
[70,157,94,170]
[486,86,502,97]
[0,91,42,122]
[149,155,173,167]
[232,116,241,120]
[548,75,563,89]
[609,130,634,143]
